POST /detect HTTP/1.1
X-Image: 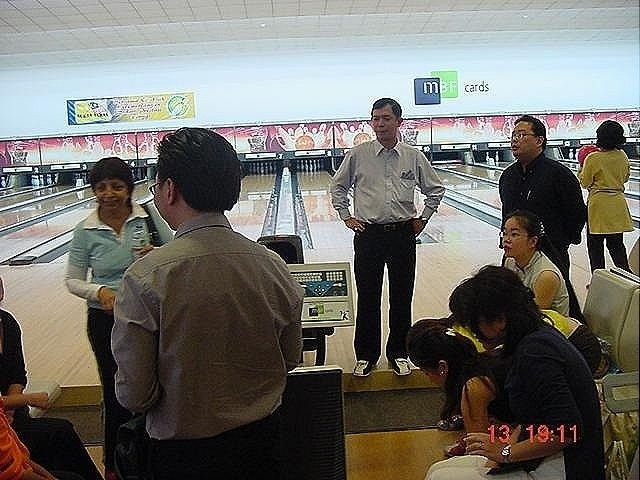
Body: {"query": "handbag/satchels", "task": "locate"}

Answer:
[501,443,511,462]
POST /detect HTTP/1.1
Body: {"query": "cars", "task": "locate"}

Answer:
[303,351,318,367]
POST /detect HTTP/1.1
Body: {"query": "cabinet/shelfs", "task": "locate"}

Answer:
[130,221,148,262]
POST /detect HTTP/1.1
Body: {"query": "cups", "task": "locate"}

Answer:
[509,133,537,140]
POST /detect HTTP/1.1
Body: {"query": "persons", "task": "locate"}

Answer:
[499,210,573,314]
[1,278,104,479]
[0,396,59,480]
[329,98,446,377]
[578,120,635,273]
[416,264,608,479]
[405,308,601,423]
[498,114,589,316]
[110,126,306,478]
[65,155,179,479]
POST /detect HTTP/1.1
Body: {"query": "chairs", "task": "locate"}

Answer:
[352,360,376,377]
[388,358,412,376]
[437,414,464,431]
[445,433,466,456]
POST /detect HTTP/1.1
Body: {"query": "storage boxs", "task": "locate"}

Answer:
[581,269,640,386]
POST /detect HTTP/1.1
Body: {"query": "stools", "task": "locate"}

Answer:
[365,221,407,232]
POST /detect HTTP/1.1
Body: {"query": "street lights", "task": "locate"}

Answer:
[114,416,145,480]
